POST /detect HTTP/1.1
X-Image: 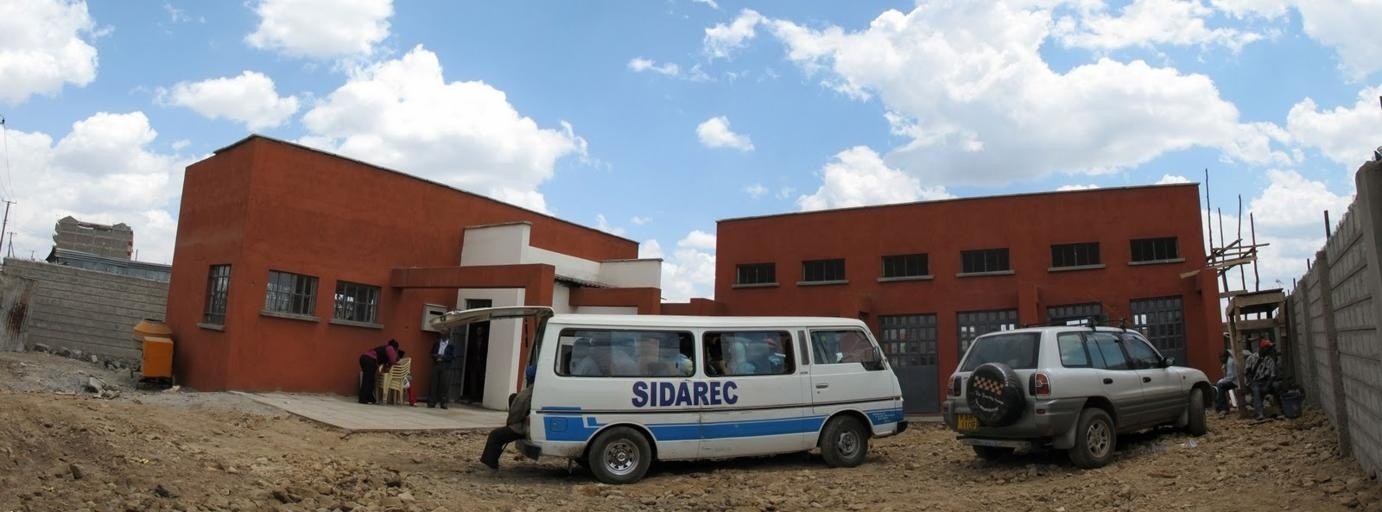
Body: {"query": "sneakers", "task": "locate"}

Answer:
[409,403,417,406]
[474,467,500,477]
[359,398,376,404]
[427,402,447,409]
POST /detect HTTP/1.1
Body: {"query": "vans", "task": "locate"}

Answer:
[430,306,904,485]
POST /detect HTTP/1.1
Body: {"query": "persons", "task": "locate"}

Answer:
[357,339,399,403]
[565,334,786,376]
[428,327,456,408]
[479,365,537,469]
[1242,338,1286,421]
[397,349,419,408]
[464,326,488,406]
[1213,349,1239,418]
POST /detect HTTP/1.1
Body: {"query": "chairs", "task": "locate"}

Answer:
[374,356,412,405]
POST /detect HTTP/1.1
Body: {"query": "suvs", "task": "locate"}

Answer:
[943,327,1212,470]
